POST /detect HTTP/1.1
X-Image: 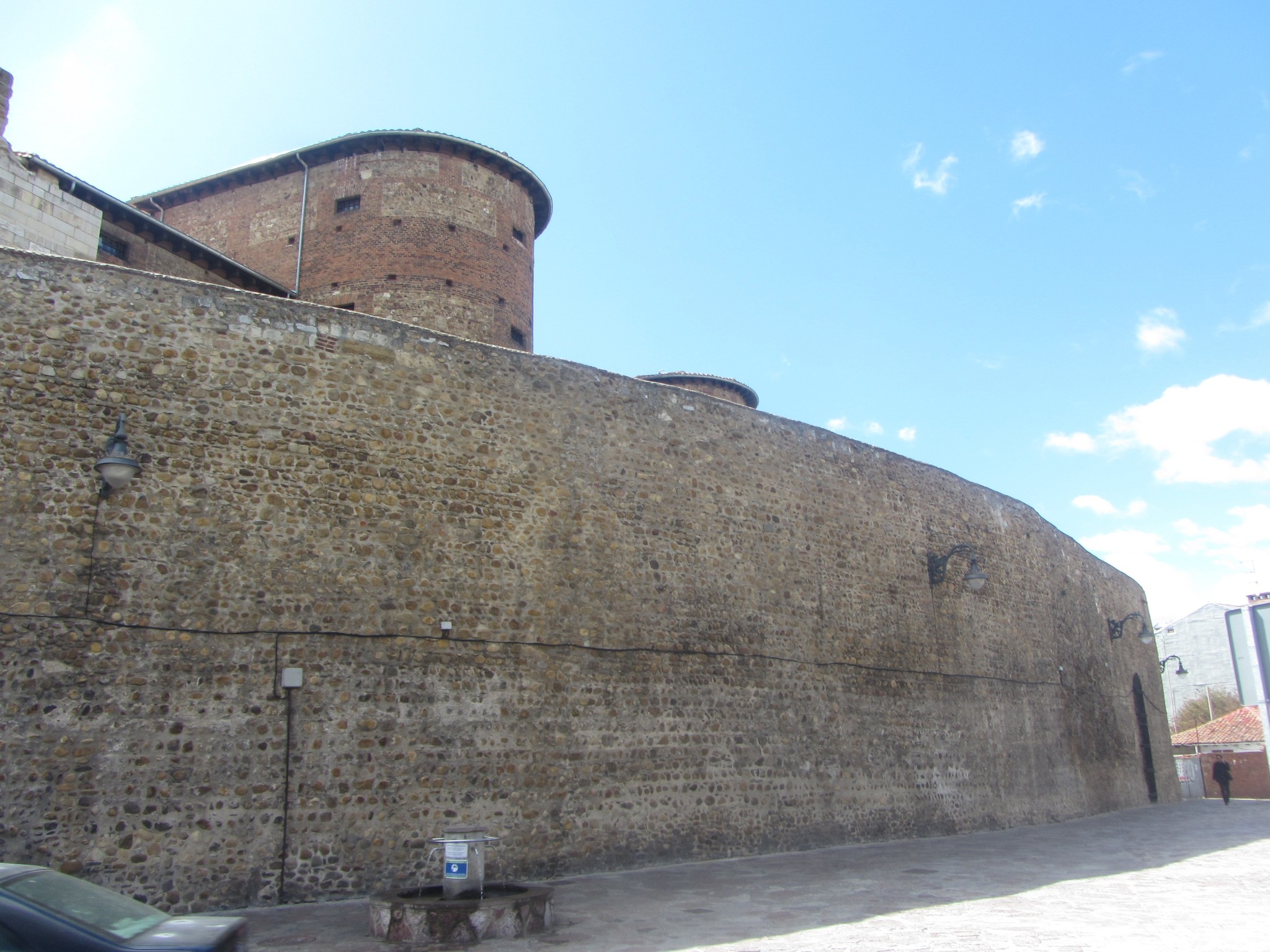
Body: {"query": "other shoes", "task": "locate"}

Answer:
[1226,799,1229,805]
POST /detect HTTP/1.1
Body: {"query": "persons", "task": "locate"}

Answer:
[1213,755,1231,806]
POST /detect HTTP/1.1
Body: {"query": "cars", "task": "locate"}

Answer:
[0,862,248,952]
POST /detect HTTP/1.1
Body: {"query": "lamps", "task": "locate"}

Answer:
[927,543,988,590]
[1159,655,1189,679]
[93,413,143,499]
[1107,613,1155,645]
